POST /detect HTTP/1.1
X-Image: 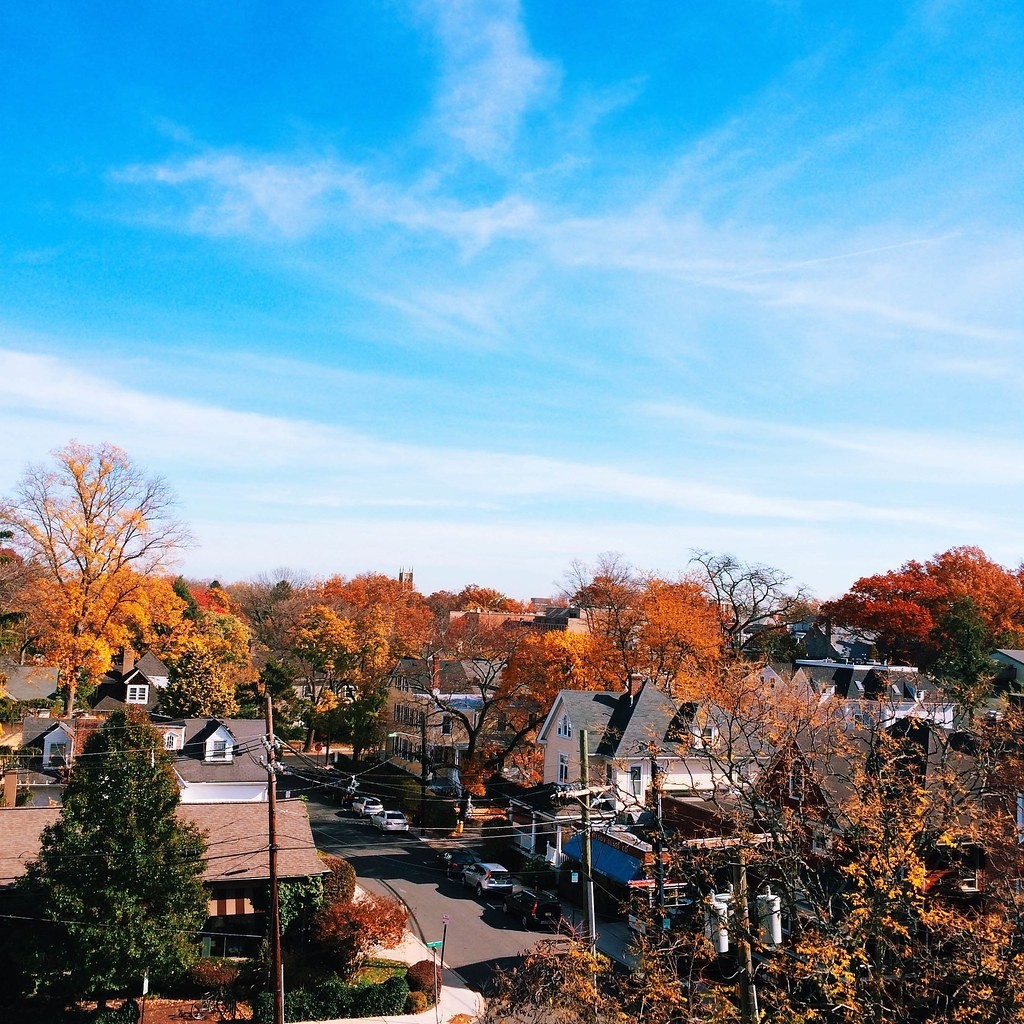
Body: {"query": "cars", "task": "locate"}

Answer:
[438,848,477,879]
[370,810,409,836]
[320,778,359,807]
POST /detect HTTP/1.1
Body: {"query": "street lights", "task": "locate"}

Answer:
[388,732,427,836]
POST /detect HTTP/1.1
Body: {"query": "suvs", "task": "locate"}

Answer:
[460,862,513,898]
[503,889,562,930]
[352,795,384,819]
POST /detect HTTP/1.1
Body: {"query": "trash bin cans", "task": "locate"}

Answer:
[456,822,464,832]
